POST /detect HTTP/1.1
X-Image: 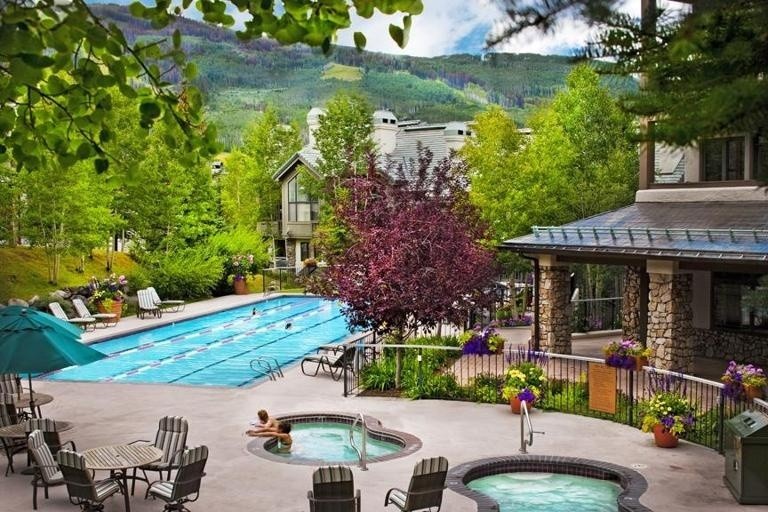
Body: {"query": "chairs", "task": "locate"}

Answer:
[384,456,448,511]
[314,343,375,359]
[48,301,95,336]
[135,288,161,322]
[147,285,185,313]
[300,346,355,383]
[73,298,118,330]
[305,465,362,512]
[0,372,209,512]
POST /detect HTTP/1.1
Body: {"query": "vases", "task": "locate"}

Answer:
[604,352,649,371]
[740,379,766,398]
[232,275,246,295]
[651,424,677,448]
[508,395,533,414]
[95,299,122,323]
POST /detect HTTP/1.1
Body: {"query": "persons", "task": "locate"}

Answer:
[285,322,293,331]
[245,421,293,453]
[252,311,259,316]
[252,307,259,312]
[249,409,280,429]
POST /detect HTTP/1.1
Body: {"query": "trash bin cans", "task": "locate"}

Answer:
[723,406,768,505]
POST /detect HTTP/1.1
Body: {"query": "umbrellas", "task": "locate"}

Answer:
[0,305,83,339]
[0,308,110,419]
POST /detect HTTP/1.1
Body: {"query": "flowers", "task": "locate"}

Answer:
[634,364,698,437]
[720,361,766,398]
[459,326,505,355]
[87,272,130,304]
[498,357,551,403]
[601,337,653,357]
[229,252,256,280]
